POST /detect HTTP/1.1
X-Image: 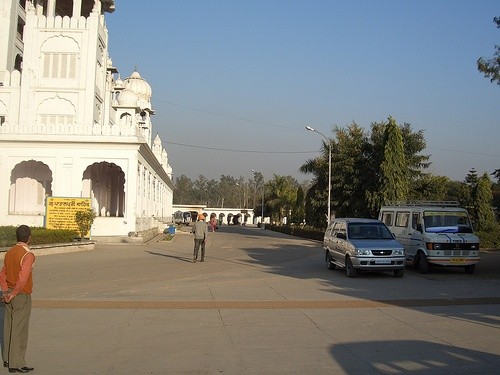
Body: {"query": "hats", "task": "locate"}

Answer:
[198,214,205,219]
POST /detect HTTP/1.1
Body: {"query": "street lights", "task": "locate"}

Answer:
[305,126,331,223]
[251,169,264,228]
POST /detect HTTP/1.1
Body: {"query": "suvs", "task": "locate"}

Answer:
[322,217,406,278]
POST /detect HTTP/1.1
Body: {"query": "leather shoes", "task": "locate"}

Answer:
[4,361,9,367]
[9,367,34,373]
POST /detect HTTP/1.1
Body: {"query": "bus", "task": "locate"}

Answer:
[378,200,480,274]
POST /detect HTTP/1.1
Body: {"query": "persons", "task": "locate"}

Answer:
[192,214,207,263]
[210,215,217,233]
[0,225,35,373]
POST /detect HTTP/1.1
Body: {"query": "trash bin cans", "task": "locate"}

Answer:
[261,224,265,229]
[167,225,175,234]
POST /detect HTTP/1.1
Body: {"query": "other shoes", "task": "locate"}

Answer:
[201,259,204,262]
[193,259,196,263]
